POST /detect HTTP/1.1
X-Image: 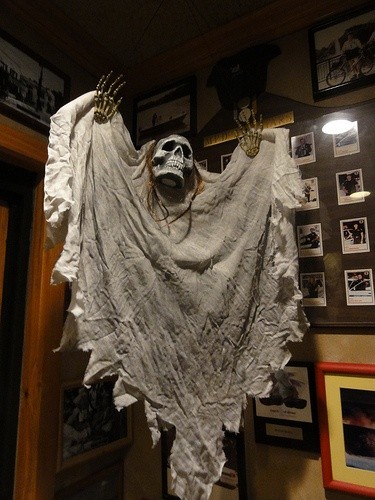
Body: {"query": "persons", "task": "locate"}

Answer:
[302,277,323,299]
[295,137,312,158]
[224,156,232,170]
[339,29,375,79]
[304,182,311,203]
[307,228,320,248]
[17,80,56,115]
[342,173,360,196]
[347,271,372,291]
[343,220,367,244]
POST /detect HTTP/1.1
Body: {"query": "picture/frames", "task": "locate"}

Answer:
[309,3,375,102]
[160,426,247,500]
[132,73,197,149]
[253,360,320,455]
[0,28,71,138]
[54,376,132,473]
[313,360,375,498]
